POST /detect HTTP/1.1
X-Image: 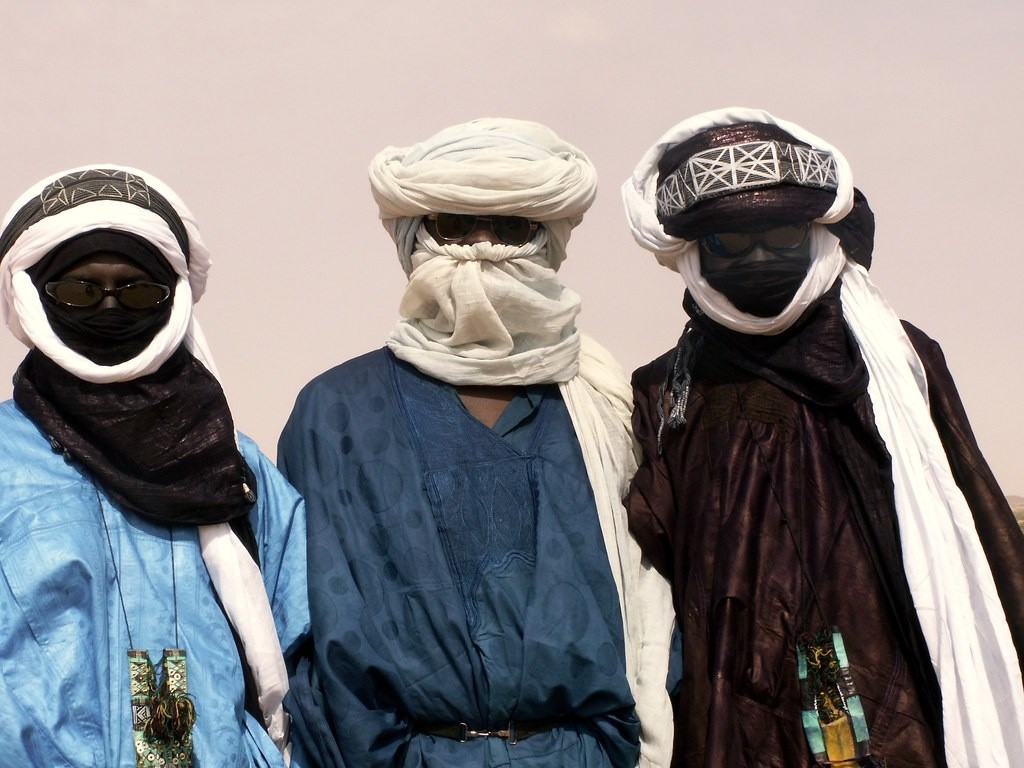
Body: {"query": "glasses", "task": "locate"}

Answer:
[43,278,173,312]
[700,220,812,257]
[421,211,540,247]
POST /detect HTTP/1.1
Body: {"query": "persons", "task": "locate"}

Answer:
[0,162,311,768]
[275,117,683,768]
[621,108,1024,768]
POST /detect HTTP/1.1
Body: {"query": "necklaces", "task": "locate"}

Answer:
[91,473,197,768]
[735,383,872,768]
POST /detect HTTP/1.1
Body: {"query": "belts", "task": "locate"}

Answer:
[396,702,573,745]
